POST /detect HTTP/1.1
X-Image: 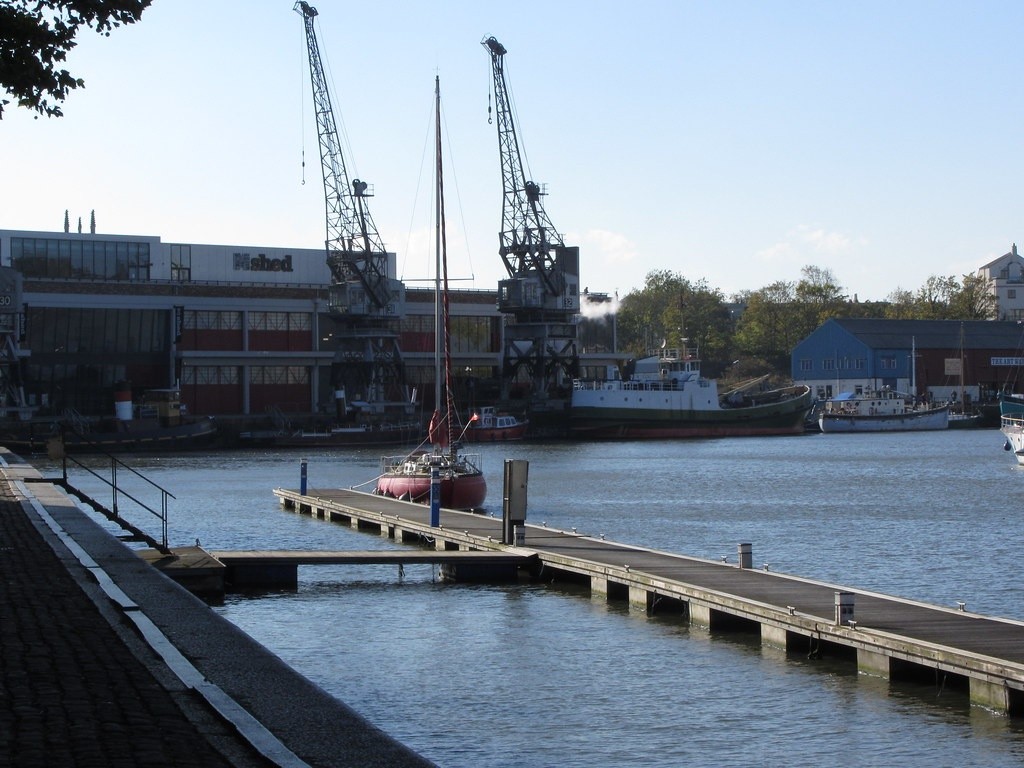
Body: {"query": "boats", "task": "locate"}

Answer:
[0,399,223,459]
[811,331,951,432]
[364,68,491,514]
[455,404,533,441]
[940,322,985,427]
[231,379,432,449]
[561,345,816,440]
[994,318,1024,469]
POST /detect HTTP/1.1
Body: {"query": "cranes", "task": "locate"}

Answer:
[285,1,425,429]
[469,26,589,417]
